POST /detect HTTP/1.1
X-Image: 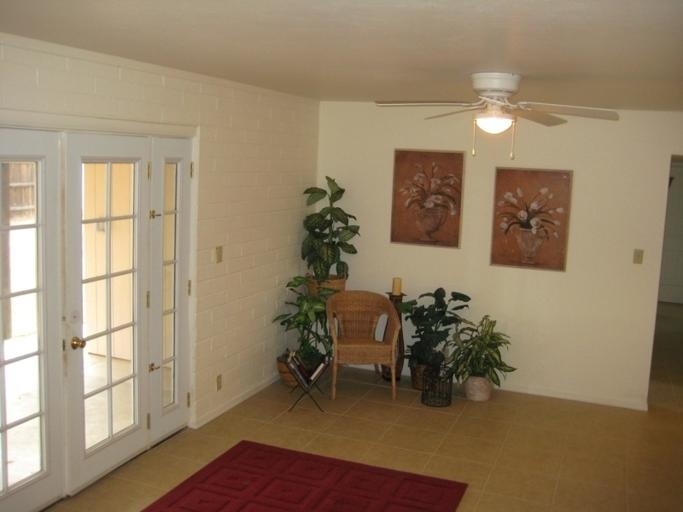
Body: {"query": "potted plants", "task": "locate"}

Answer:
[395,286,517,404]
[273,175,360,387]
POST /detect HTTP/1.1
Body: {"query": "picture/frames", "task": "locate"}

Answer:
[390,149,575,273]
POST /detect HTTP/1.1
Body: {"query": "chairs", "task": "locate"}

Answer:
[325,290,402,405]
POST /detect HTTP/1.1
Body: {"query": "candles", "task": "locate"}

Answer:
[392,278,401,296]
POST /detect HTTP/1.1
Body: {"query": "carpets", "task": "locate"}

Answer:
[133,440,469,511]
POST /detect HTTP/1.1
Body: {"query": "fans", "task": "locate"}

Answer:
[372,72,621,129]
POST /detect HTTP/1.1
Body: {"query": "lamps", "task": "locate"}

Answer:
[471,109,517,134]
